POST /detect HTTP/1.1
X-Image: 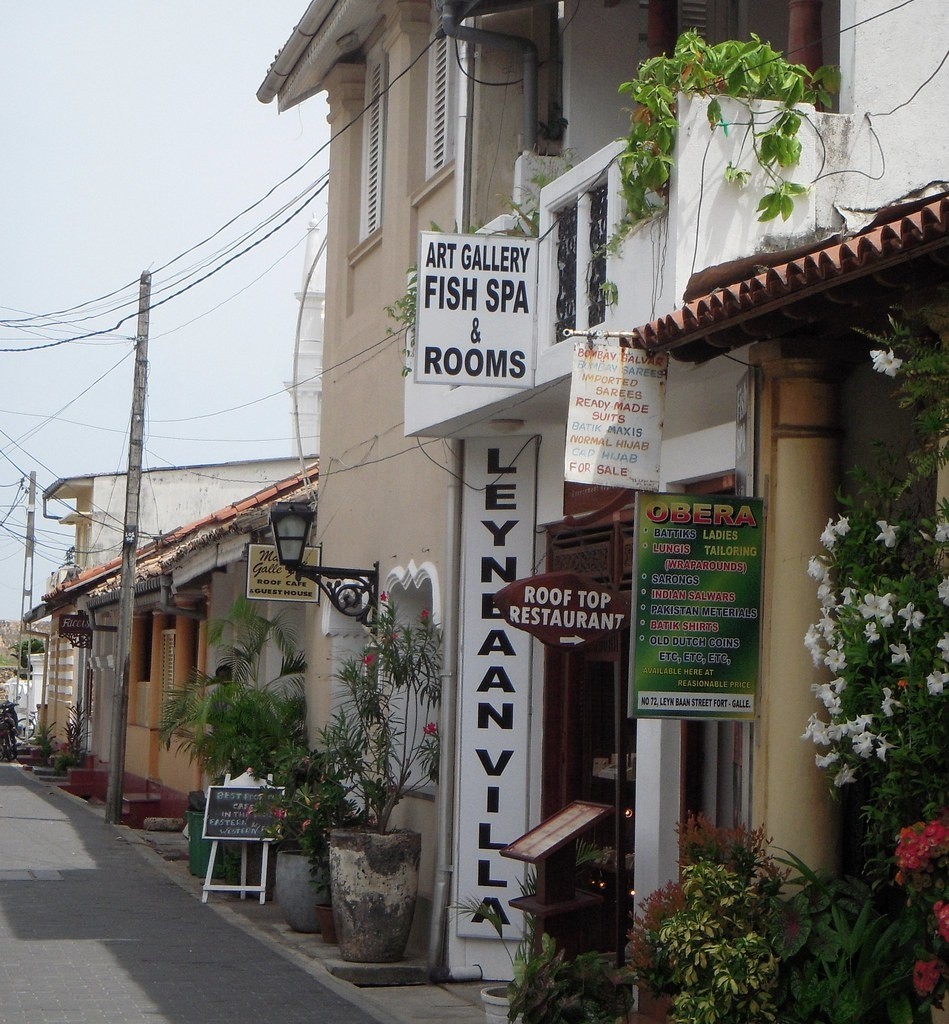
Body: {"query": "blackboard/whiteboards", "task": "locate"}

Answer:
[202,783,285,842]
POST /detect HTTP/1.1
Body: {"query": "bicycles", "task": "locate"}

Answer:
[16,710,40,741]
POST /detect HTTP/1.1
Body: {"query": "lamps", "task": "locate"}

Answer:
[270,503,313,562]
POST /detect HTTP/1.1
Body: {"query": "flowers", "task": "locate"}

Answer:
[324,592,443,829]
[801,305,948,1011]
[249,751,377,852]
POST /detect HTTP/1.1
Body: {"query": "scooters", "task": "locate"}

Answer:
[0,700,19,761]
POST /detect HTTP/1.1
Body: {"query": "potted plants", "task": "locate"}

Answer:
[306,854,336,945]
[157,595,307,901]
[448,842,604,1023]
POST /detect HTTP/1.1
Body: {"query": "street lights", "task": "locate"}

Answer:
[263,495,385,639]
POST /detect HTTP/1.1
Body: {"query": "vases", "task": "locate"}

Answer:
[274,853,329,932]
[330,827,421,962]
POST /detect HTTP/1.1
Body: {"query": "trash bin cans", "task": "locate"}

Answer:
[186,809,225,879]
